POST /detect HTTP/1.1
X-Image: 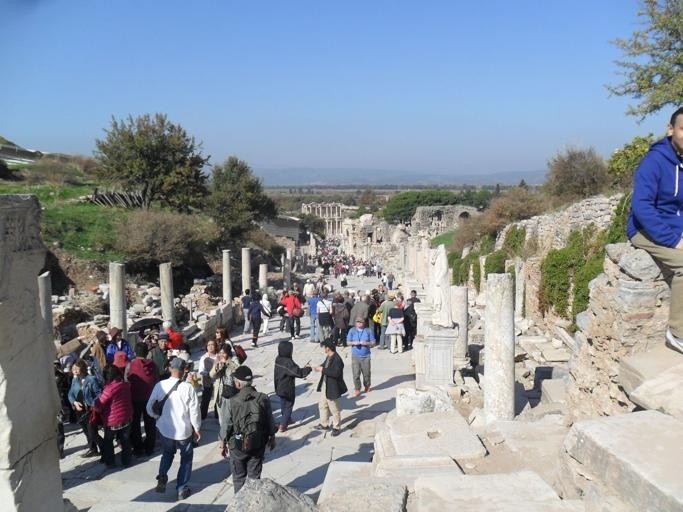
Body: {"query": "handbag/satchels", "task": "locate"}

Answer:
[152,399,162,416]
[292,308,305,317]
[229,340,248,364]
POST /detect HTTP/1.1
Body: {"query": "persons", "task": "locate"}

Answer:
[219,365,276,493]
[315,337,347,436]
[627,107,683,354]
[146,358,202,501]
[241,289,273,347]
[303,273,420,354]
[54,324,192,468]
[199,327,242,445]
[346,314,376,397]
[273,341,313,432]
[302,238,382,279]
[277,290,305,340]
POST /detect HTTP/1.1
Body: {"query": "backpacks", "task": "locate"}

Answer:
[231,389,266,454]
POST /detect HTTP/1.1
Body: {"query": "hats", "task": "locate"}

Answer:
[170,357,186,369]
[156,331,172,342]
[231,365,253,381]
[356,316,366,323]
[388,291,395,299]
[112,350,131,369]
[109,326,124,340]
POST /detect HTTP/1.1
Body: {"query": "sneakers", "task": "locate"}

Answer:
[314,424,329,430]
[156,479,167,493]
[364,386,370,392]
[331,429,340,436]
[177,487,191,500]
[664,326,683,354]
[354,389,360,397]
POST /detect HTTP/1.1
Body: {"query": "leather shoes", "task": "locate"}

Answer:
[83,448,99,457]
